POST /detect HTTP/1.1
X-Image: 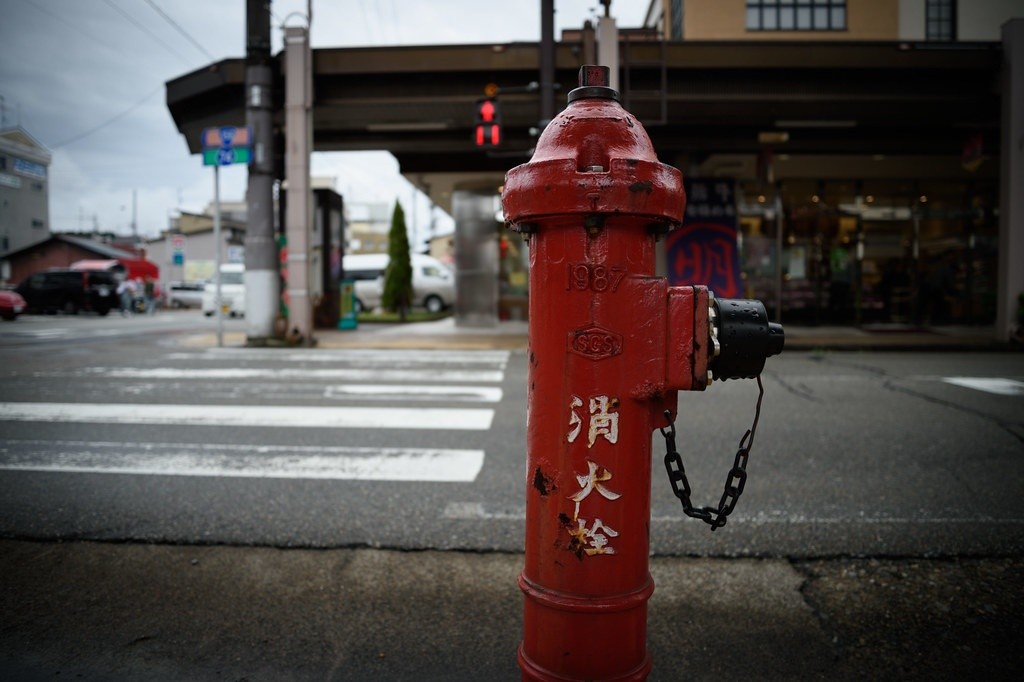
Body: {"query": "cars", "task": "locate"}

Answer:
[202,262,248,321]
[163,280,207,310]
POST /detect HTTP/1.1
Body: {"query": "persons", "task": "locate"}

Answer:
[115,272,158,317]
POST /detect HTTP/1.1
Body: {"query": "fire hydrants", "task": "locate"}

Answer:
[499,65,786,682]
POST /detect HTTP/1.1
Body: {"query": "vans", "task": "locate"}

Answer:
[343,253,458,317]
[12,268,120,316]
